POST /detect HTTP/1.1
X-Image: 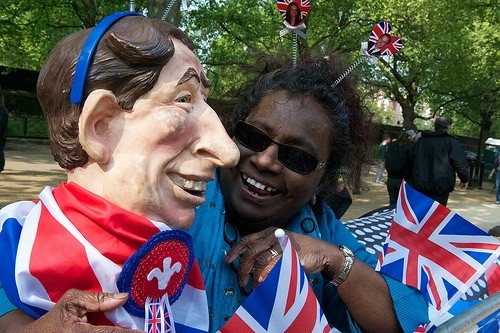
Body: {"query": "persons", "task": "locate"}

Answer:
[368,34,391,55]
[488,155,500,204]
[0,49,430,333]
[0,15,240,332]
[413,117,468,207]
[384,128,416,211]
[286,5,300,26]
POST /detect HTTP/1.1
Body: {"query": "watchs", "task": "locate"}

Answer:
[329,245,354,287]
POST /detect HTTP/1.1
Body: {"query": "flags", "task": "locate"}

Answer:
[214,237,330,333]
[375,177,500,312]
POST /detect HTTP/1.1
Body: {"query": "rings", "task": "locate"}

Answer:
[269,247,279,259]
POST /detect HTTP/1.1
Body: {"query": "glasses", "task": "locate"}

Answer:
[234,120,329,176]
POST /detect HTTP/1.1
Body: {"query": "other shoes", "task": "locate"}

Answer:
[495,201,500,204]
